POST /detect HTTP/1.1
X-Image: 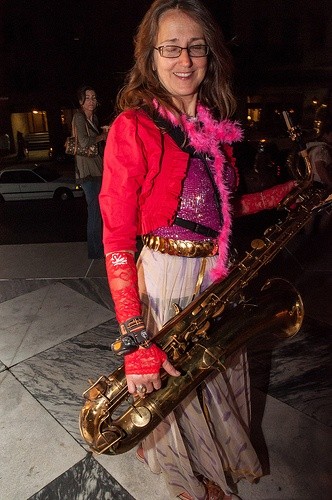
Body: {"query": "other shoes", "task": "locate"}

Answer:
[178,491,193,500]
[88,249,105,259]
[135,448,144,462]
[205,481,241,500]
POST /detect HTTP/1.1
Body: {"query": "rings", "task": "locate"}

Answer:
[137,384,148,396]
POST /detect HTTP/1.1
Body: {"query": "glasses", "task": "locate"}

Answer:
[152,44,211,58]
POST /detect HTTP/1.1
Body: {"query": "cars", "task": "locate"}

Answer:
[0,167,85,206]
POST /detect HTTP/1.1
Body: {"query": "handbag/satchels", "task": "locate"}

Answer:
[64,112,98,157]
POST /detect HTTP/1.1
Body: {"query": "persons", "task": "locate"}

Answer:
[95,1,300,499]
[1,130,11,154]
[237,99,332,235]
[16,130,26,160]
[63,85,114,261]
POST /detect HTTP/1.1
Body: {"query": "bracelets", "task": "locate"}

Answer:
[111,316,148,355]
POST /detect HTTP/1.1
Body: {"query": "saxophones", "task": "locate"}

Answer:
[79,110,330,456]
